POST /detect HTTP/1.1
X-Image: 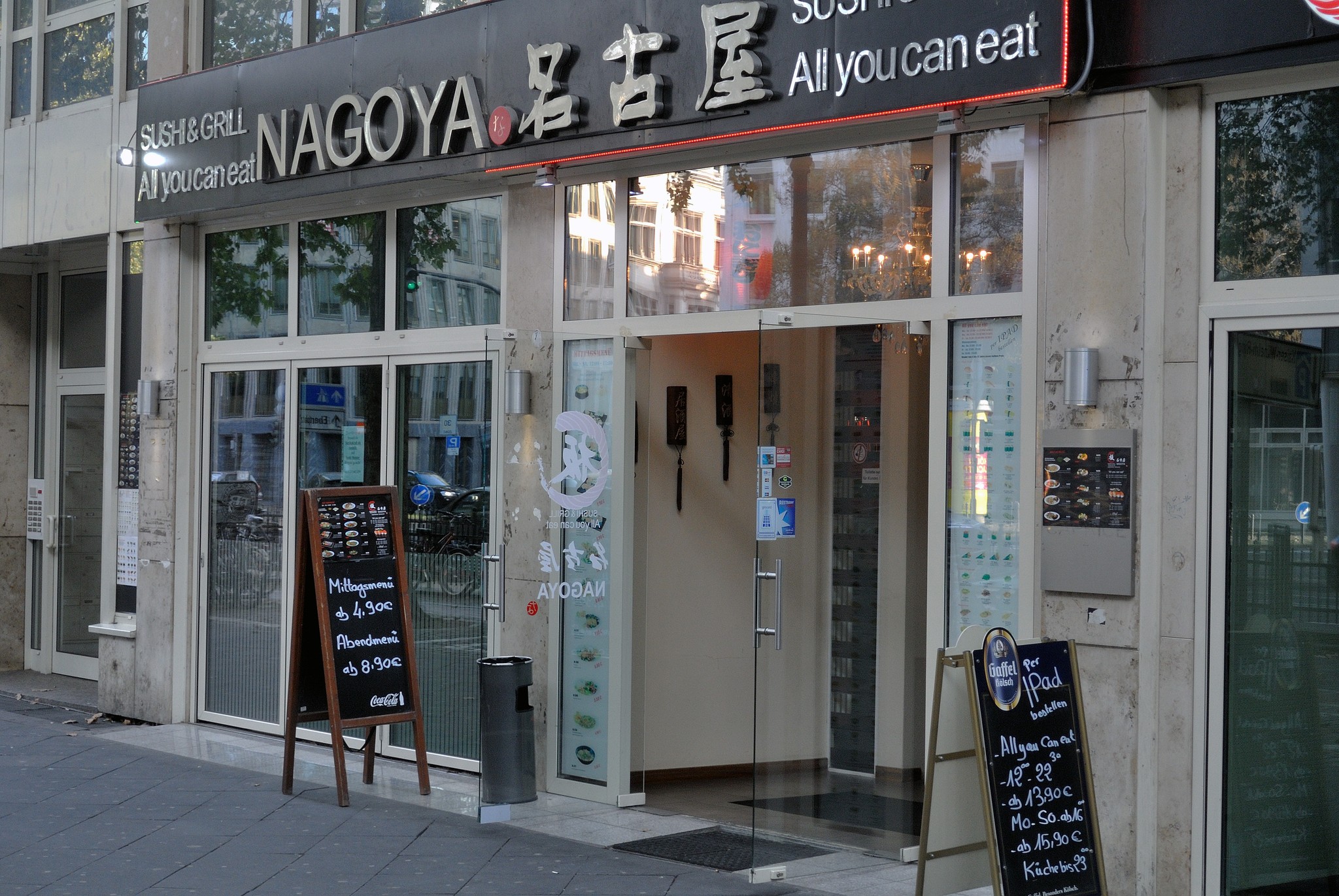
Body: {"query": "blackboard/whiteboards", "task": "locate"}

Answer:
[290,483,421,726]
[920,637,1109,896]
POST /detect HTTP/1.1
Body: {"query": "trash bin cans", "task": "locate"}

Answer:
[478,654,541,804]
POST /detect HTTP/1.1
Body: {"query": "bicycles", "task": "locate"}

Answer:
[208,496,283,610]
[397,509,484,597]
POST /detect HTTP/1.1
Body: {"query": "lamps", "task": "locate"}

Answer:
[1063,348,1099,406]
[116,130,140,168]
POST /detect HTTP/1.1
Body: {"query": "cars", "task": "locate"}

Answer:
[405,469,491,554]
